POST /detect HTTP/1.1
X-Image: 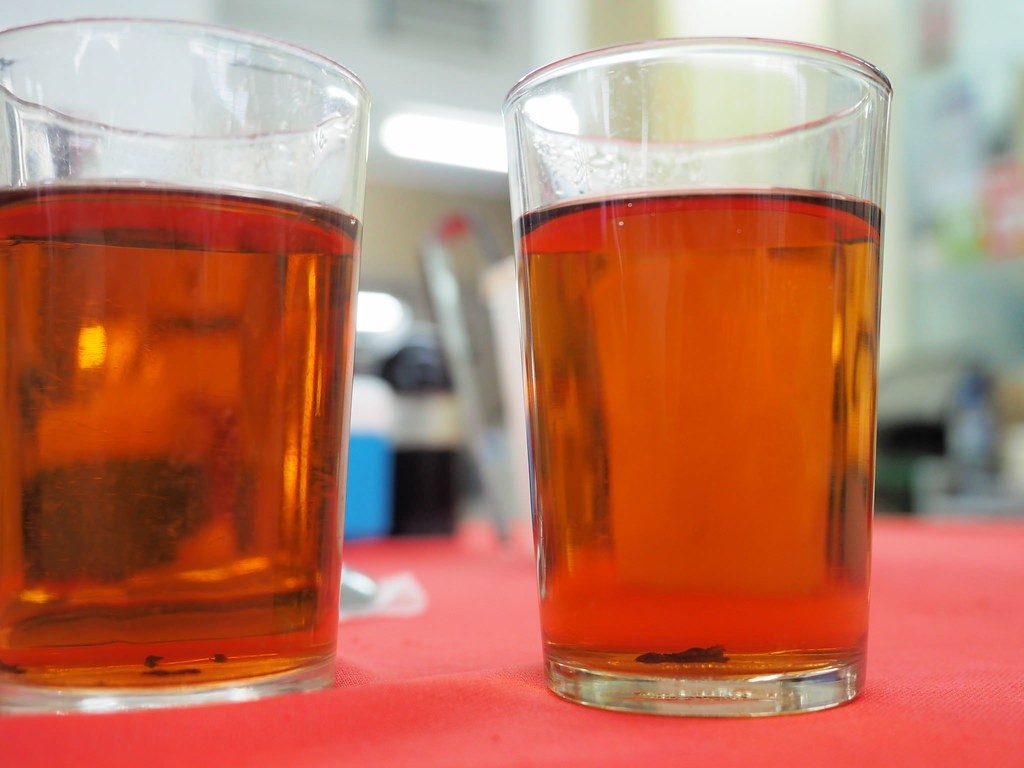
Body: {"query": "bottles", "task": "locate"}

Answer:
[944,375,1002,494]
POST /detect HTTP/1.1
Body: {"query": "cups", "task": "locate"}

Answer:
[501,37,893,715]
[0,19,371,711]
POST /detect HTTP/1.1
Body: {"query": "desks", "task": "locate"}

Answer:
[0,515,1024,768]
